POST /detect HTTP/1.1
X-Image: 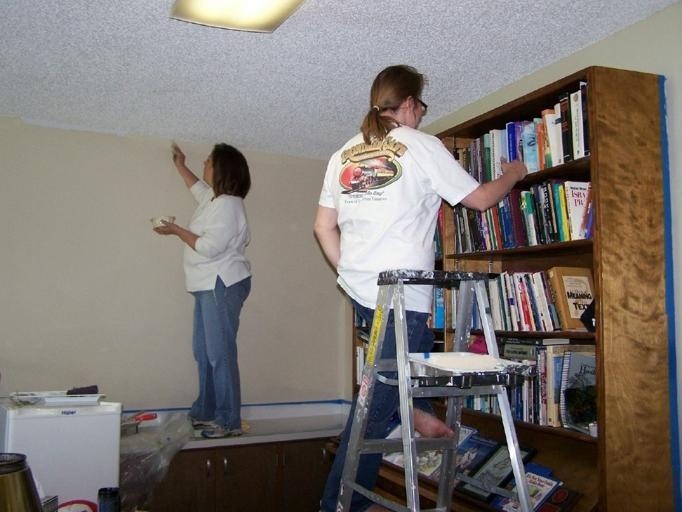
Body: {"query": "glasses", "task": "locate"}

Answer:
[418,99,427,116]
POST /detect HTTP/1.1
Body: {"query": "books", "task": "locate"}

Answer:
[444,82,590,186]
[42,393,104,406]
[452,178,594,256]
[448,268,599,332]
[424,285,445,330]
[379,418,563,512]
[356,345,369,386]
[459,333,599,428]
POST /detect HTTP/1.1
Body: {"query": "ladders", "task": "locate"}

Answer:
[337,269,534,512]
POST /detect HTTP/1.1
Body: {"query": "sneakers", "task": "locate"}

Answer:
[189,416,242,438]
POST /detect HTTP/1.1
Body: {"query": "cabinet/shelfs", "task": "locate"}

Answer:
[350,63,681,512]
[0,413,351,511]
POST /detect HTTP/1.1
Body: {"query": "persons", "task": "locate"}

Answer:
[312,63,530,508]
[151,143,253,442]
